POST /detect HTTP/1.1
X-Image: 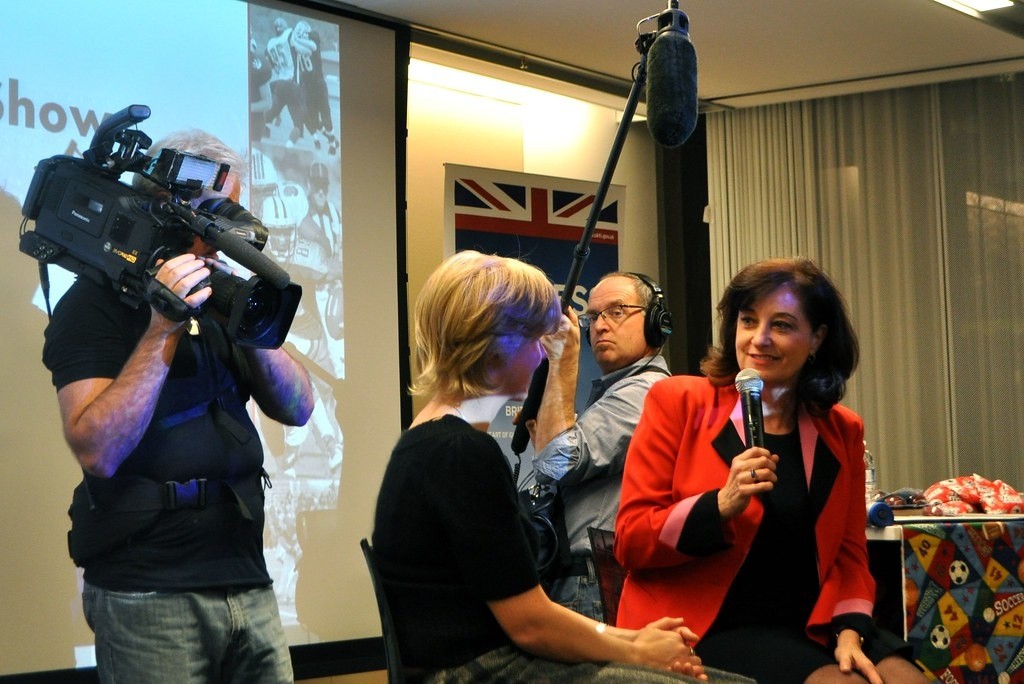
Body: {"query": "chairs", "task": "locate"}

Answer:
[588,526,627,628]
[358,537,421,684]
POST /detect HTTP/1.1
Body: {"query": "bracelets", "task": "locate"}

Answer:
[835,623,864,647]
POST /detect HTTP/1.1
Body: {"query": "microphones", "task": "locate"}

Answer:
[191,215,292,291]
[645,0,699,148]
[735,368,764,450]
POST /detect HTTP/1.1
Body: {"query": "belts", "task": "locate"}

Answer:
[551,562,589,579]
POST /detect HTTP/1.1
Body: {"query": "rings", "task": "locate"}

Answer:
[750,468,757,482]
[688,644,695,655]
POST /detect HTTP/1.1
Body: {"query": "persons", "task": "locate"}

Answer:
[612,256,931,684]
[512,270,673,627]
[247,16,341,628]
[42,128,315,684]
[371,249,759,684]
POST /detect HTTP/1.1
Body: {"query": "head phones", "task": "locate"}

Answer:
[585,274,674,348]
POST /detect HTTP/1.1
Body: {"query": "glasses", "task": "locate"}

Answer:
[576,303,647,328]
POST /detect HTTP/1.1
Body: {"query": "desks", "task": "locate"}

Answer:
[866,520,1023,684]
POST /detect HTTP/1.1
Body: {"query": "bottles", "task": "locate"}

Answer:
[863,440,877,513]
